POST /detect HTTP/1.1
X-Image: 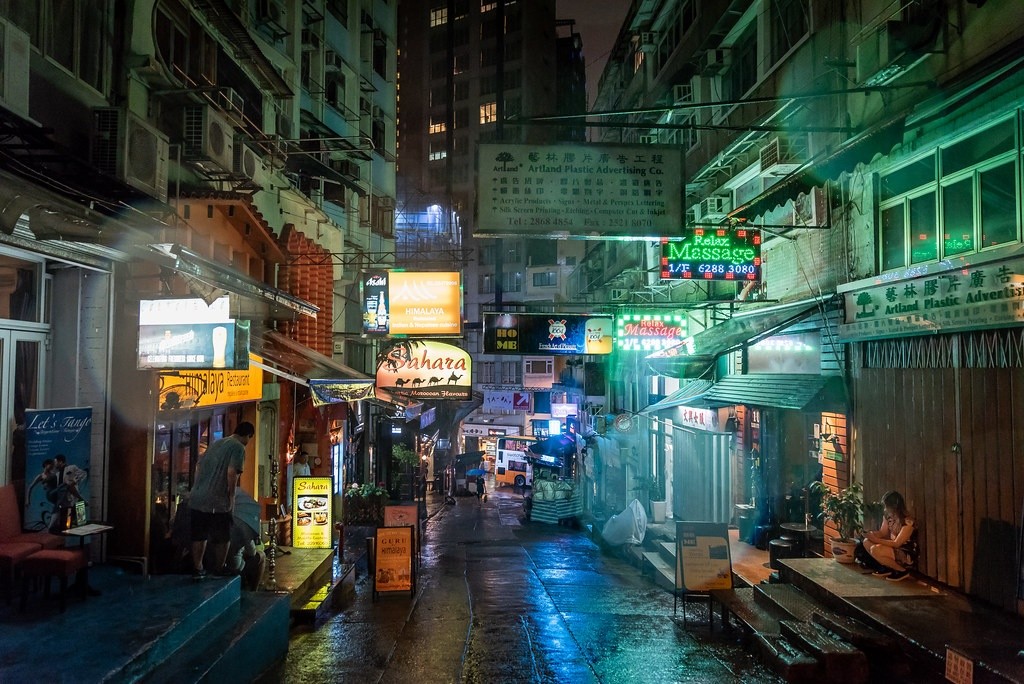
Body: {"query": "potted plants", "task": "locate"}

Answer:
[624,469,667,524]
[811,480,867,564]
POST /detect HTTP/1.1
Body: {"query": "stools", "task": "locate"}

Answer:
[740,516,824,569]
[28,548,82,612]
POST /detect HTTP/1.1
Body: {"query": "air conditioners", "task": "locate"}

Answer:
[374,106,386,121]
[666,85,695,116]
[221,87,244,118]
[327,53,343,72]
[336,158,360,181]
[179,102,235,179]
[225,143,267,191]
[85,107,170,212]
[0,12,45,145]
[268,114,290,160]
[637,31,659,55]
[759,134,809,177]
[699,47,732,74]
[359,97,371,116]
[698,197,731,222]
[855,17,908,88]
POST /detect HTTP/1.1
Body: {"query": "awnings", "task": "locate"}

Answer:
[455,451,486,469]
[633,299,832,416]
[131,242,320,317]
[250,320,424,415]
[300,109,374,162]
[253,133,366,199]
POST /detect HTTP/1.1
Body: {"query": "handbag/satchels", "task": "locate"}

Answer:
[484,495,487,503]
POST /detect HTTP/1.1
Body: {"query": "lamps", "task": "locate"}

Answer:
[724,406,742,433]
[819,418,837,444]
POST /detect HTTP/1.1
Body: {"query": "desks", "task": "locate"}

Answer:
[780,522,817,532]
[259,513,292,524]
[53,520,114,581]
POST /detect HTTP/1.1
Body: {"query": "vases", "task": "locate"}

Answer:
[343,497,381,526]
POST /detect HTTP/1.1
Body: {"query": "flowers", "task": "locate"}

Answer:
[342,481,391,498]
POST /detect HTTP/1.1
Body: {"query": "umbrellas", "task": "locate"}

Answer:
[465,468,486,483]
[233,489,262,545]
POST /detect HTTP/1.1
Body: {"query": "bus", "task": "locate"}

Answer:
[494,434,577,491]
[523,411,567,438]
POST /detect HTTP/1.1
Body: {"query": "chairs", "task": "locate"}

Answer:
[0,484,64,550]
[0,542,42,601]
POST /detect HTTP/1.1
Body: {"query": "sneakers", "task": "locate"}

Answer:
[192,568,223,580]
[870,568,910,582]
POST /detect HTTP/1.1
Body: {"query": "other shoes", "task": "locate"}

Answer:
[78,498,89,507]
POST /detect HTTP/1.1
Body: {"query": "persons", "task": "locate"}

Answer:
[293,452,310,476]
[25,454,89,534]
[862,491,919,582]
[475,475,487,507]
[186,421,255,580]
[226,540,267,593]
